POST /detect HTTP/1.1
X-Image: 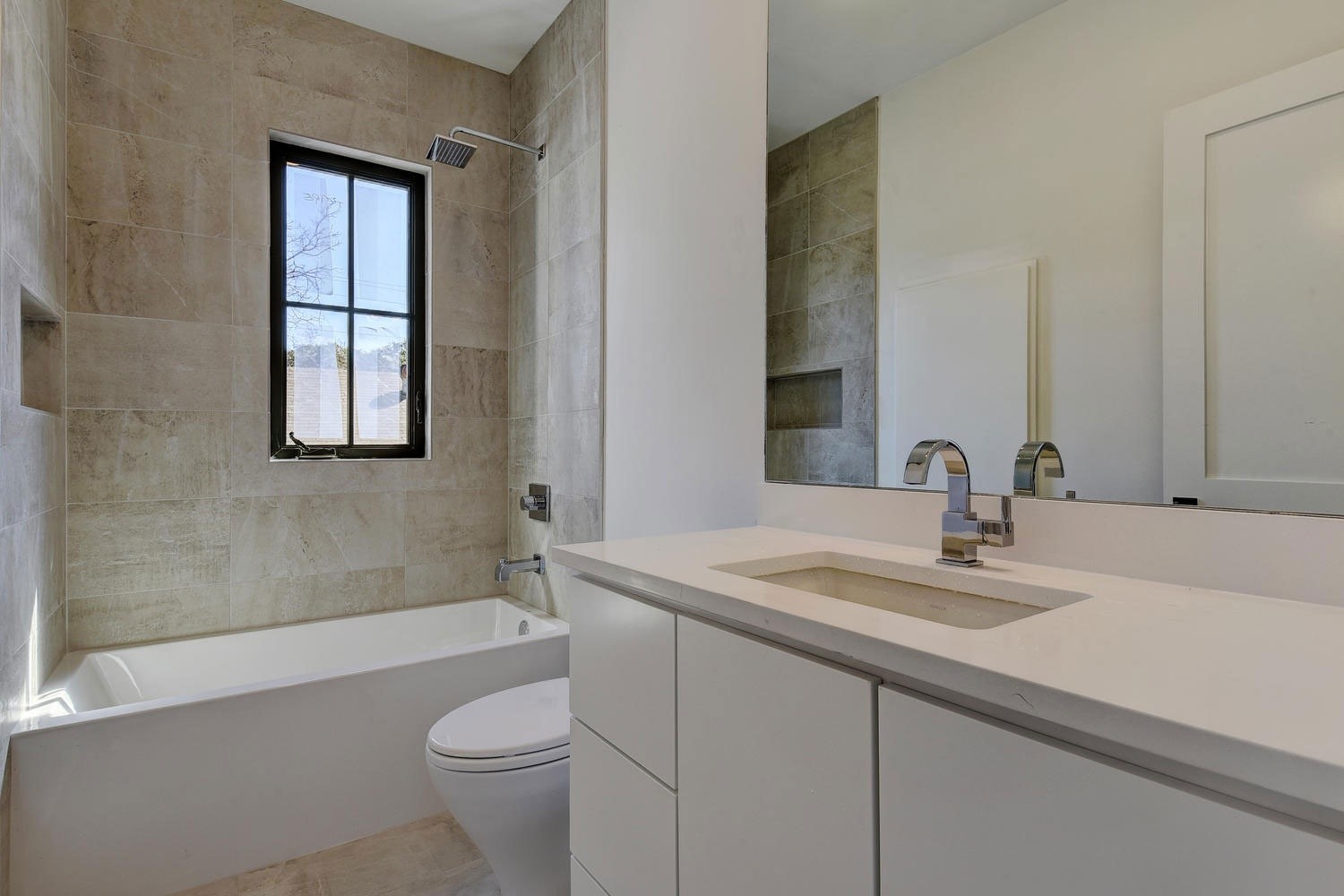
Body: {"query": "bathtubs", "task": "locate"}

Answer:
[12,592,571,896]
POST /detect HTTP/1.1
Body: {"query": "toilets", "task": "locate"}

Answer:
[425,679,573,896]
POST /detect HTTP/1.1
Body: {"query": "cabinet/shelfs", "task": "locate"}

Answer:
[568,575,1343,896]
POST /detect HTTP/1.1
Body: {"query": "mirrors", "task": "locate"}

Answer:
[765,0,1344,523]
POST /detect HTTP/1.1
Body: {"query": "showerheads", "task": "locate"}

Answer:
[425,125,545,169]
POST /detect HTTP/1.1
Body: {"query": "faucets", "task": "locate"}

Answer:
[903,438,1016,568]
[1013,439,1078,503]
[493,553,546,582]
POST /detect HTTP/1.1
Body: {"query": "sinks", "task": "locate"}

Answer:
[708,549,1093,636]
